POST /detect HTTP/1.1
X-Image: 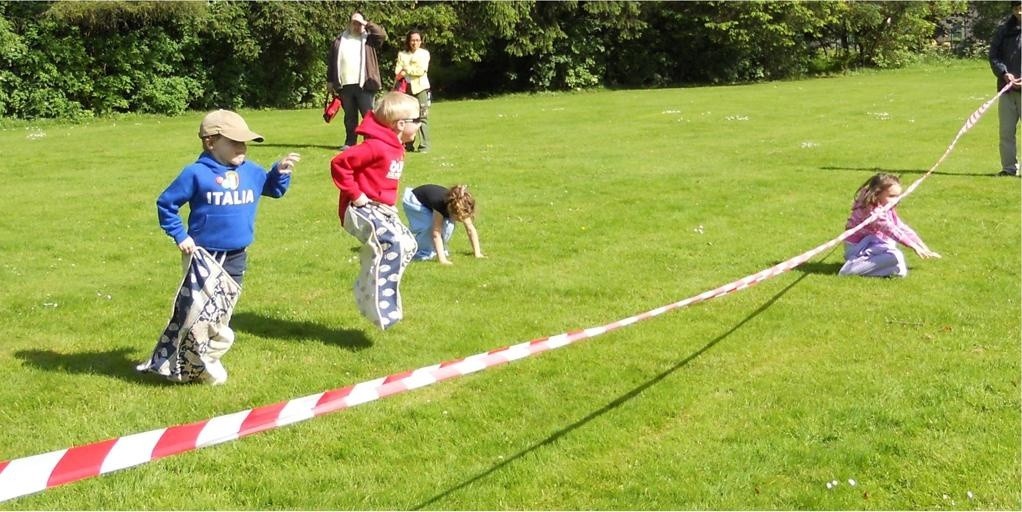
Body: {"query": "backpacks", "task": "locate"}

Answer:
[393,70,407,94]
[324,89,342,124]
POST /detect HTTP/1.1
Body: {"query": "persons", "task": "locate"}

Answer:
[329,91,428,331]
[326,13,387,149]
[134,108,300,386]
[401,181,485,265]
[839,176,942,277]
[394,31,430,154]
[989,0,1021,177]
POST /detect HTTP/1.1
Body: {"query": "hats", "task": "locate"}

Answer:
[198,108,263,143]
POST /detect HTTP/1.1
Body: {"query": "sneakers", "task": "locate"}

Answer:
[998,171,1010,175]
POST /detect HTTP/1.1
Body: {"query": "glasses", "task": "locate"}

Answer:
[392,117,421,127]
